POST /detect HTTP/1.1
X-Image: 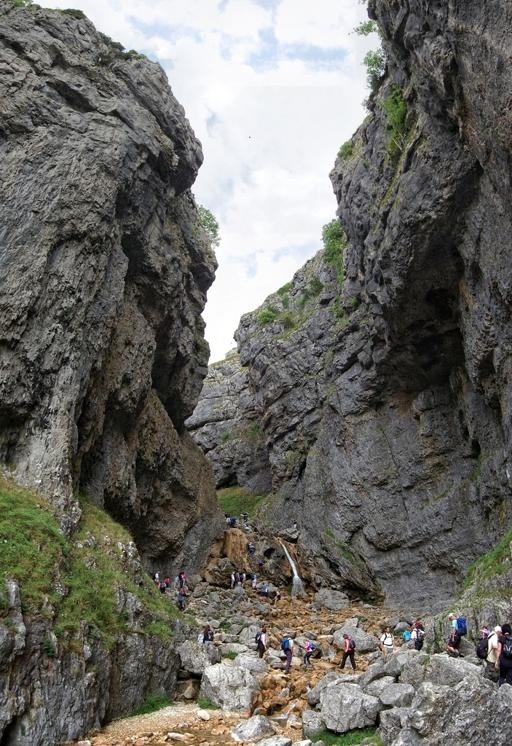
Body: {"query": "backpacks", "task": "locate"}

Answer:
[477,635,497,658]
[503,634,511,655]
[457,618,467,636]
[349,640,356,648]
[310,643,315,650]
[281,639,289,651]
[255,633,262,643]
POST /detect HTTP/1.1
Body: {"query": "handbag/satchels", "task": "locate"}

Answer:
[415,641,423,649]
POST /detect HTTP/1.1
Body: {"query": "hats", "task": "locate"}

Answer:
[495,626,502,633]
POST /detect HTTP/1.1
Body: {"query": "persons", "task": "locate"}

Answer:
[303,639,314,667]
[153,570,188,610]
[258,560,264,575]
[338,634,356,671]
[230,569,257,588]
[247,541,256,555]
[257,629,267,658]
[403,618,425,651]
[479,621,512,686]
[446,613,464,657]
[379,626,394,656]
[269,559,273,570]
[197,624,215,647]
[280,636,293,674]
[224,511,247,528]
